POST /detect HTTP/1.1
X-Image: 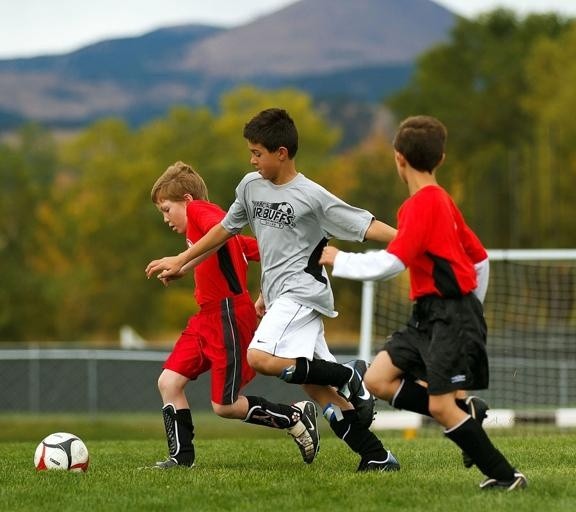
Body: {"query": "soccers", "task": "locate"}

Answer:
[33,431,89,473]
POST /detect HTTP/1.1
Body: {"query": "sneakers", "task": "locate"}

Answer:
[356,451,399,476]
[479,469,529,493]
[463,396,488,468]
[289,400,320,464]
[137,460,196,471]
[336,359,376,429]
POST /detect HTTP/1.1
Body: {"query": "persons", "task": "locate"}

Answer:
[150,161,319,474]
[319,115,527,494]
[145,108,399,473]
